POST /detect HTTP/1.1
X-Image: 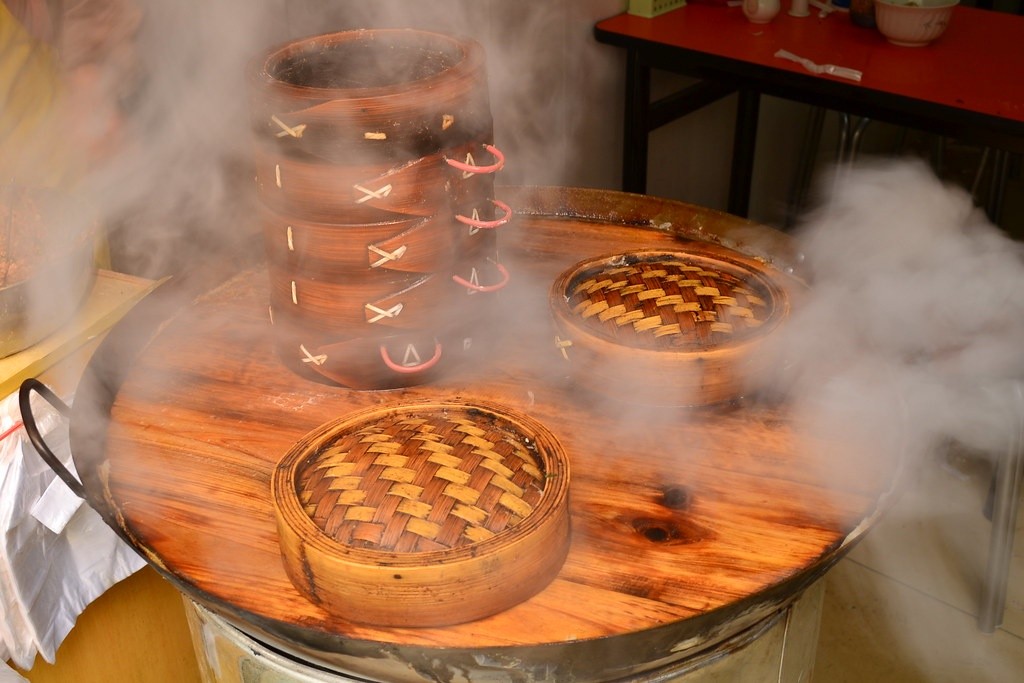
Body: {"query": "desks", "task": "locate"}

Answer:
[594,0,1024,220]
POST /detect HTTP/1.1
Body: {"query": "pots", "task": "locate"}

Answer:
[16,180,913,683]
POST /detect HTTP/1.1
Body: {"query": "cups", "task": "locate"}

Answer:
[831,0,851,13]
[848,0,876,28]
[741,0,782,24]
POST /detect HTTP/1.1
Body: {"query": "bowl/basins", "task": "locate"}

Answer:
[0,176,95,360]
[873,0,961,48]
